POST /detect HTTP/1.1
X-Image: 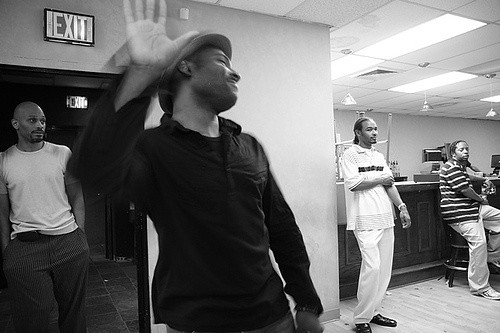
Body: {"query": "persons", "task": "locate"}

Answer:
[0,101,88,333]
[438,140,500,300]
[340,117,411,333]
[77,0,328,333]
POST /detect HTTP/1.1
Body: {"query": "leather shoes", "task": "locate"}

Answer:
[370,314,397,327]
[355,323,372,333]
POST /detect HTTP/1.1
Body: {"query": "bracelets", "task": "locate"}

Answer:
[296,304,319,317]
[480,197,484,203]
[398,203,406,210]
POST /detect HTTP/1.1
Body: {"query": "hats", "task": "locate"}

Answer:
[157,30,231,113]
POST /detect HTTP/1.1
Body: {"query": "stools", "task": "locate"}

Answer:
[443,227,469,288]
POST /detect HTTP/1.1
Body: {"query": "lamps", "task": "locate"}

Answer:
[340,49,357,105]
[484,74,500,117]
[418,62,435,112]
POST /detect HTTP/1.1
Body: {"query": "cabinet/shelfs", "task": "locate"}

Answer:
[337,186,500,301]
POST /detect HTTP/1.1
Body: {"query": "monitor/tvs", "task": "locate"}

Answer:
[422,148,441,163]
[491,155,500,168]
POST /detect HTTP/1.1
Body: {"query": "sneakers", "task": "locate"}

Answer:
[471,283,500,300]
[492,260,500,267]
